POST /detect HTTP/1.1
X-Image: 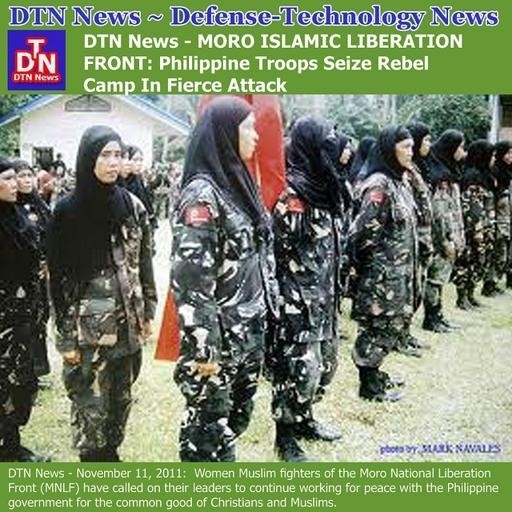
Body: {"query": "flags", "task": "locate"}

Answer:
[152,94,288,363]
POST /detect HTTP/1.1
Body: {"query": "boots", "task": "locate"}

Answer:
[457,288,489,314]
[0,433,121,463]
[295,418,345,442]
[276,418,307,462]
[359,361,406,403]
[392,326,430,357]
[482,280,510,298]
[423,306,462,334]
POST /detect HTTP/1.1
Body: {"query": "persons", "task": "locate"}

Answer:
[259,115,512,464]
[170,92,280,463]
[42,123,158,464]
[0,141,191,462]
